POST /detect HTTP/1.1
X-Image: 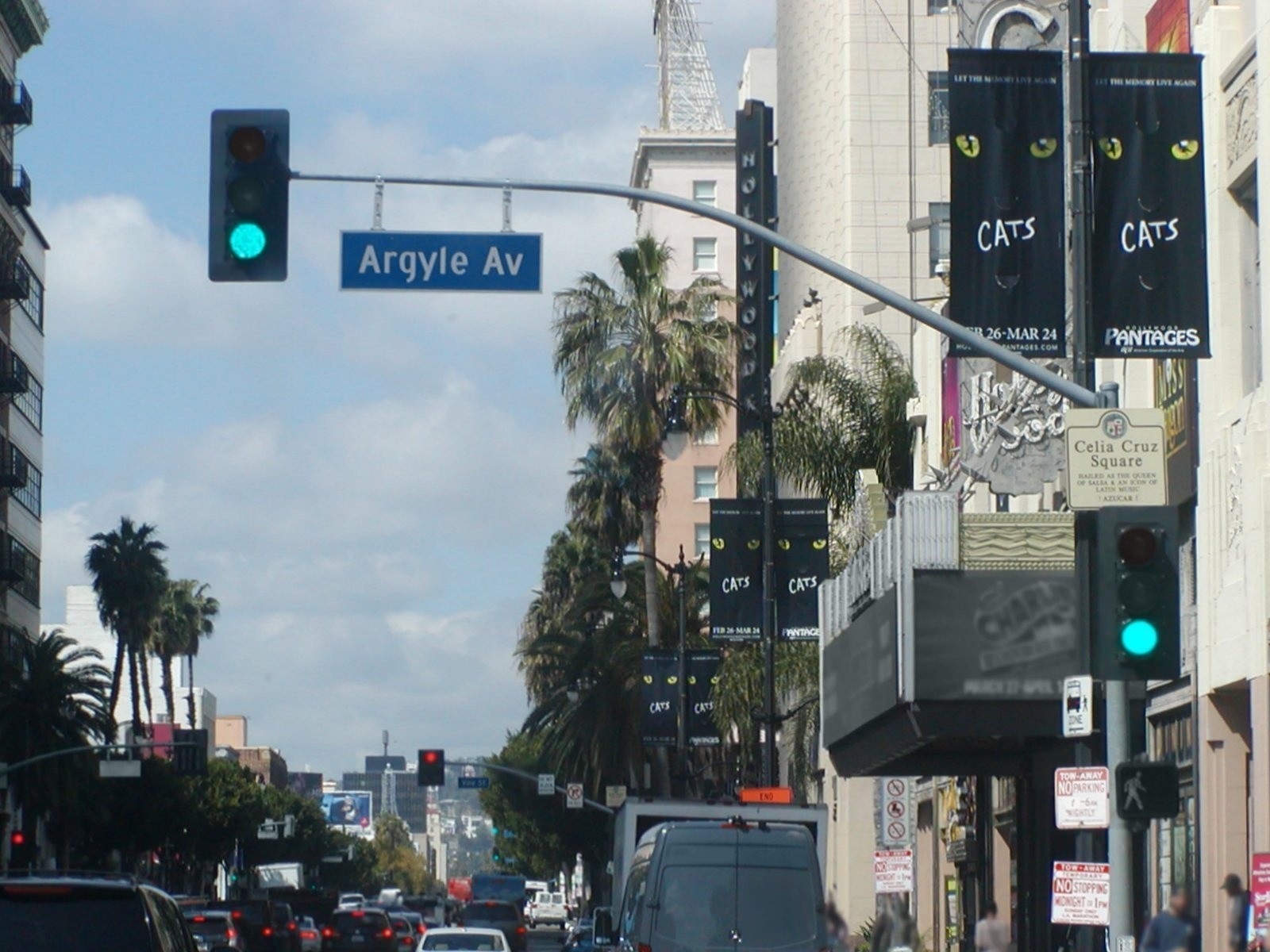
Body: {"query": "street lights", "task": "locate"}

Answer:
[608,542,707,799]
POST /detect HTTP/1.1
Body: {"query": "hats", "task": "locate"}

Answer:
[1220,874,1242,891]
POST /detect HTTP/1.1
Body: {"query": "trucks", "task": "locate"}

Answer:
[471,871,527,914]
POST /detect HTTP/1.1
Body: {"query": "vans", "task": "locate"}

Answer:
[525,881,550,921]
[591,815,827,951]
[524,891,571,929]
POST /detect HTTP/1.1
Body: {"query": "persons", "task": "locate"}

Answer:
[1139,885,1202,952]
[974,901,1010,952]
[1221,875,1250,952]
[824,903,848,952]
[871,892,918,952]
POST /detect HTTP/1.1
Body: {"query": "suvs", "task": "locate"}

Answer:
[0,869,199,952]
[464,898,529,952]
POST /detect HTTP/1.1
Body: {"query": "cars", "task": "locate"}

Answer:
[417,927,515,952]
[565,926,605,952]
[370,890,463,952]
[340,893,365,908]
[323,906,399,952]
[172,895,321,951]
[182,910,242,951]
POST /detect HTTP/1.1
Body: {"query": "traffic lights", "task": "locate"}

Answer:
[1113,764,1180,824]
[417,748,447,787]
[9,831,31,878]
[205,107,297,286]
[492,846,500,862]
[1095,503,1184,683]
